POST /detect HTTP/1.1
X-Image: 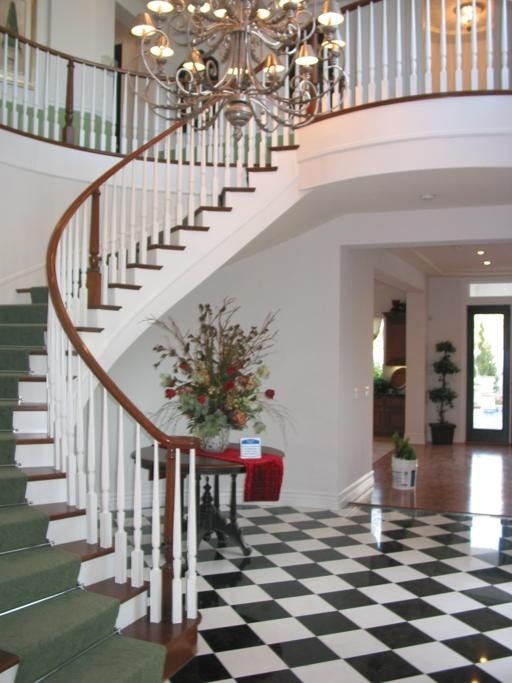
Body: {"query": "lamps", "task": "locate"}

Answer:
[453,2,484,34]
[123,0,350,144]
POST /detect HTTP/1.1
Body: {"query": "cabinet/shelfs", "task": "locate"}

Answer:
[374,393,405,438]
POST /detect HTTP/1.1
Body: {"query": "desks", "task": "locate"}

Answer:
[129,442,286,571]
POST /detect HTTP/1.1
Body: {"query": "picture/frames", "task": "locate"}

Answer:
[0,0,37,92]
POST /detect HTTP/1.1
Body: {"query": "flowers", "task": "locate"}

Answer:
[136,293,294,440]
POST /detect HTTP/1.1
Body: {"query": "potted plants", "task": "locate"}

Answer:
[475,321,497,392]
[426,340,463,445]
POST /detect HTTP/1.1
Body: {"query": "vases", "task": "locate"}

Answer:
[198,422,230,455]
